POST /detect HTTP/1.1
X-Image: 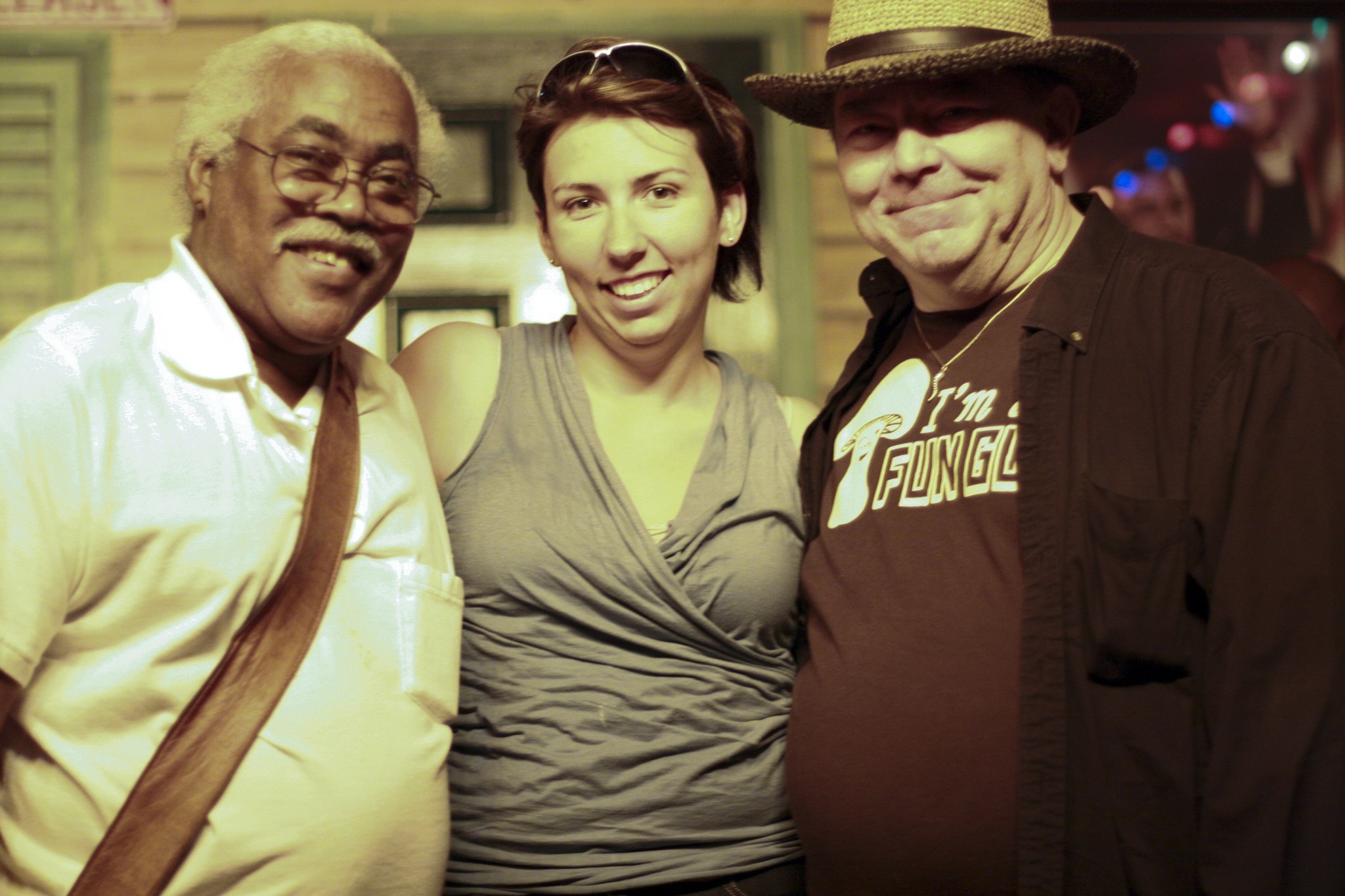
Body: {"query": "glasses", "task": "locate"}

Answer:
[535,40,724,155]
[233,135,442,227]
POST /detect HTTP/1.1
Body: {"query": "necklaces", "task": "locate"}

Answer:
[912,278,1040,401]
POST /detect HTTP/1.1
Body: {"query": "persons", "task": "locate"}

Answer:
[1,21,462,895]
[392,34,806,896]
[744,1,1345,895]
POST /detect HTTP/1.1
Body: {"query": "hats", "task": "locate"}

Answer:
[743,0,1138,133]
